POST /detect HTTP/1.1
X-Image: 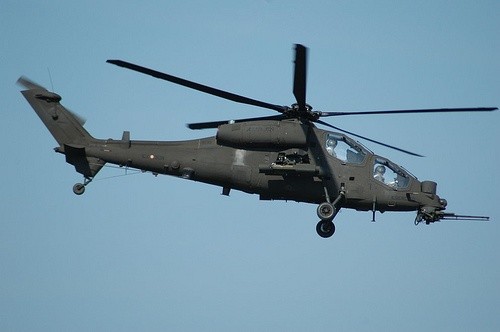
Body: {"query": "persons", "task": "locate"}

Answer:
[372,163,387,185]
[326,138,341,158]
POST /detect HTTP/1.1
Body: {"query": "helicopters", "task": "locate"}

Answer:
[16,44,500,238]
